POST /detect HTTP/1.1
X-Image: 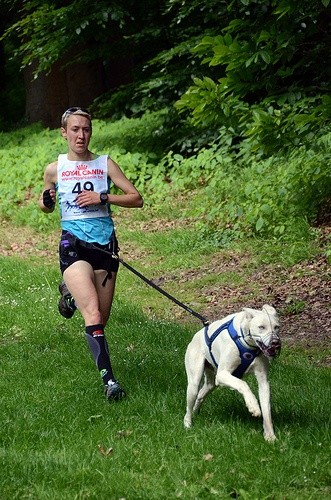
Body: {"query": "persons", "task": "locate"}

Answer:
[38,106,144,403]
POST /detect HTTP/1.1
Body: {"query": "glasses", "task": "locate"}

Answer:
[62,107,90,121]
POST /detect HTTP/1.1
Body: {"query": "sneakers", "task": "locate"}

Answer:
[59,283,78,319]
[104,382,126,403]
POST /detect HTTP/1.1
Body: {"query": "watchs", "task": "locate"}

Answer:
[99,191,108,207]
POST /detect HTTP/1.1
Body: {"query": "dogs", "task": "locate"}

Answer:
[181,301,284,446]
